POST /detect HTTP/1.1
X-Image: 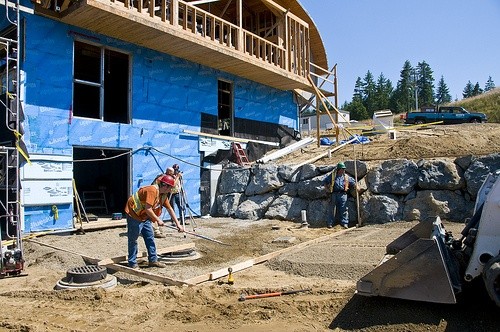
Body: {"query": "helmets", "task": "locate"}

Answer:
[337,162,346,169]
[160,175,175,186]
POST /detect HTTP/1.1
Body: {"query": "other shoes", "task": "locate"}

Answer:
[132,266,140,270]
[149,261,166,267]
[328,224,333,227]
[180,221,189,225]
[155,233,166,238]
[341,223,348,228]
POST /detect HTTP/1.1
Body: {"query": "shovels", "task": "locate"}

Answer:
[73,179,86,234]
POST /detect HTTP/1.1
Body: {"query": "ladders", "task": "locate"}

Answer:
[232,141,250,165]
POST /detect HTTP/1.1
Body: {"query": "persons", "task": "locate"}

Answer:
[323,161,360,229]
[124,175,184,271]
[150,167,182,238]
[169,164,190,226]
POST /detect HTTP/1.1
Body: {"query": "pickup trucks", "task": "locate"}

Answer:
[404,106,488,124]
